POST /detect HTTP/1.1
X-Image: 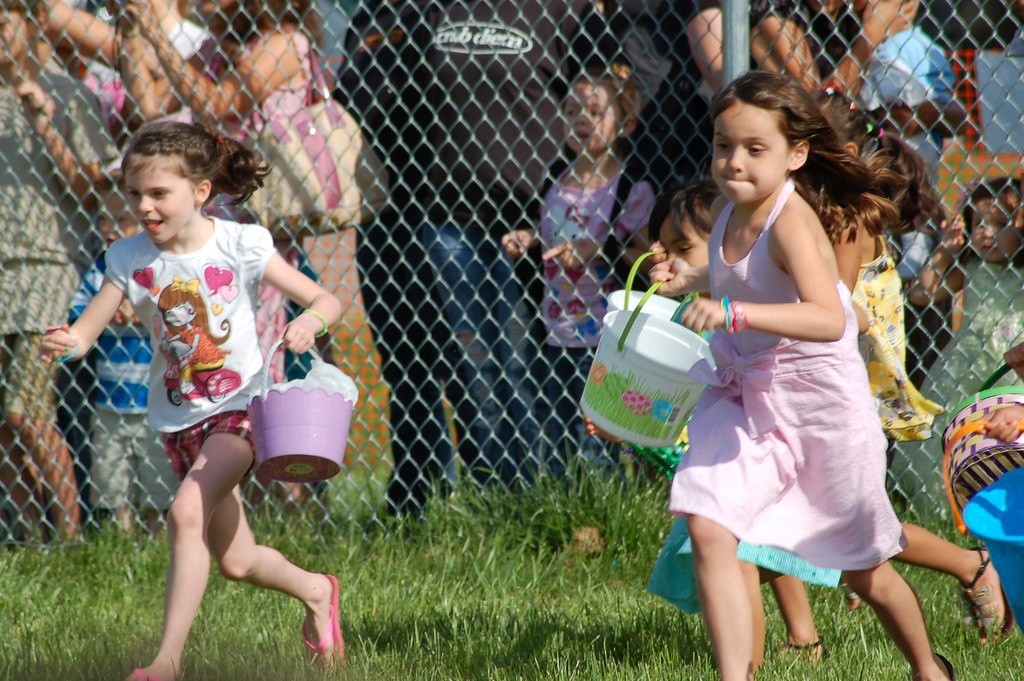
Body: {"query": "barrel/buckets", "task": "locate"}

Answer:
[580,285,716,448]
[943,421,1023,634]
[603,247,713,349]
[248,342,355,482]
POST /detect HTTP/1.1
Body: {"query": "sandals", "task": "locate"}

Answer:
[964,547,1014,646]
[787,634,830,661]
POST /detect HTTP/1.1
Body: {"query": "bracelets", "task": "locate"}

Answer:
[303,308,329,339]
[728,300,748,335]
[721,294,731,335]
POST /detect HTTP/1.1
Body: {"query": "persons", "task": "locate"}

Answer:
[39,120,345,681]
[424,0,1024,680]
[0,0,393,552]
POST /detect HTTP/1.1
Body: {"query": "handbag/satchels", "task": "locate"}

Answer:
[236,29,389,236]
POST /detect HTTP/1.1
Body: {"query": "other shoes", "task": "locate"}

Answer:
[931,652,954,681]
